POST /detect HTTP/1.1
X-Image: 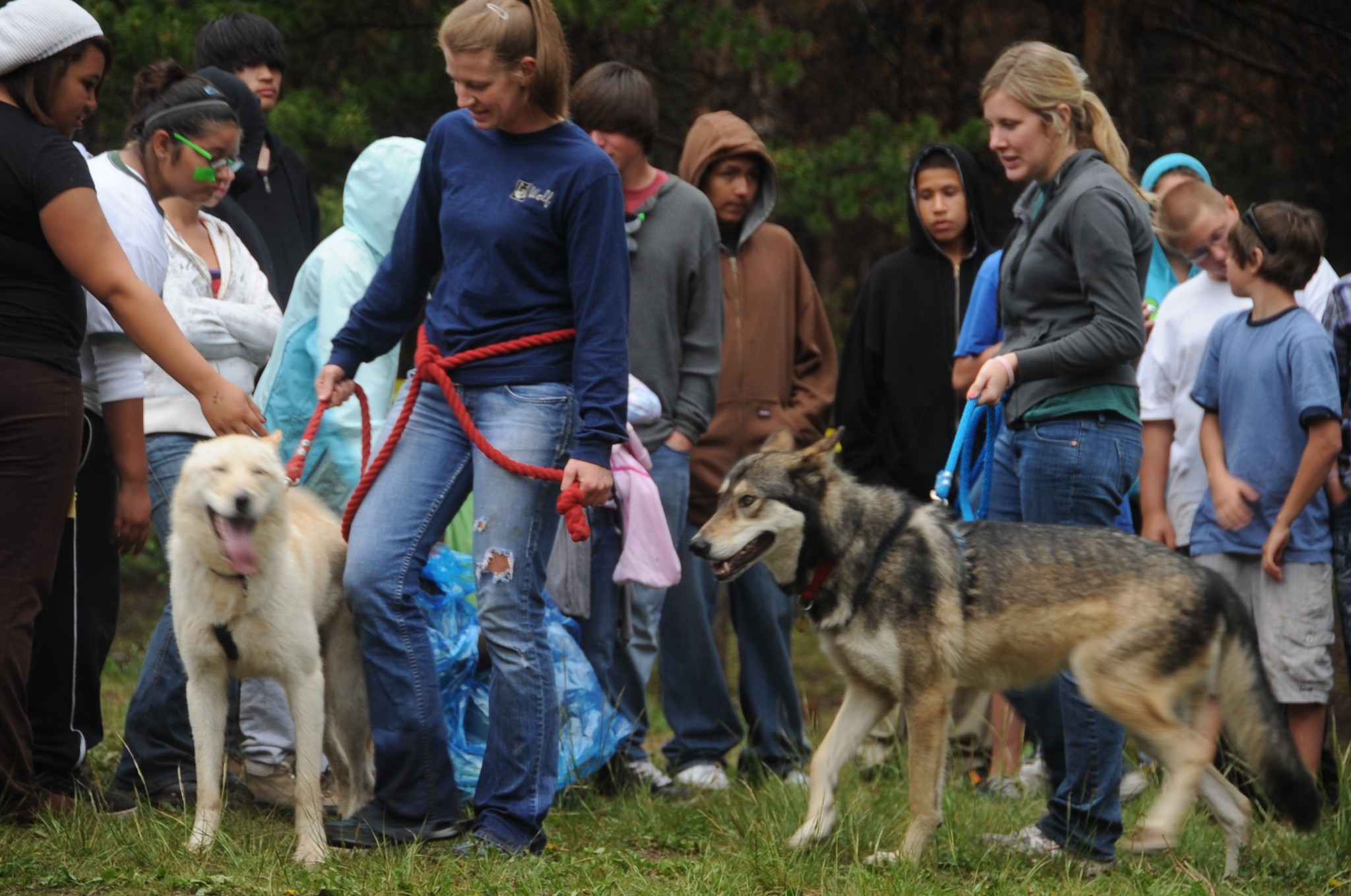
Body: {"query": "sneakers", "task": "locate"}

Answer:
[236,754,340,821]
[598,759,700,806]
[323,818,461,848]
[451,836,512,860]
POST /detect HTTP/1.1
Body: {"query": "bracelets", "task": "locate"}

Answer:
[995,354,1017,392]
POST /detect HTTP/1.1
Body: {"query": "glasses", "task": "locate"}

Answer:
[1179,207,1231,265]
[624,211,642,261]
[1241,202,1276,255]
[173,132,245,173]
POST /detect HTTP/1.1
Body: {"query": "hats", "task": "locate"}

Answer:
[0,0,104,75]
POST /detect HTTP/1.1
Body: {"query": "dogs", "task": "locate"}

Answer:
[689,425,1323,882]
[168,429,370,874]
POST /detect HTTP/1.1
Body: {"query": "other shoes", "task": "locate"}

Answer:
[983,824,1114,876]
[676,764,730,792]
[49,775,138,818]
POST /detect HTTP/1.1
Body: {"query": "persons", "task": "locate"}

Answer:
[837,142,1019,784]
[1148,197,1335,830]
[562,57,748,812]
[104,131,306,819]
[910,246,1130,803]
[314,2,632,855]
[233,131,447,787]
[0,0,272,817]
[58,56,247,768]
[1130,153,1228,336]
[1111,172,1339,554]
[958,40,1176,870]
[178,7,325,322]
[1321,270,1351,758]
[656,110,840,809]
